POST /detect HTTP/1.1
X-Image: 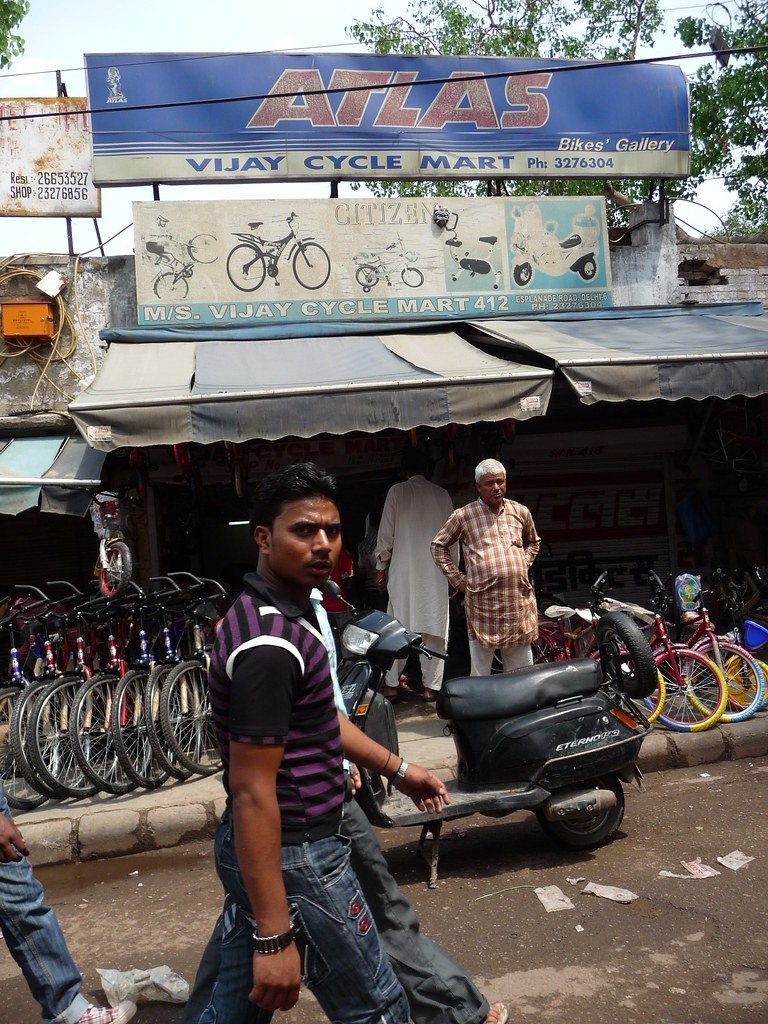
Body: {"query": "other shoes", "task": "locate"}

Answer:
[424,688,435,702]
[384,687,398,703]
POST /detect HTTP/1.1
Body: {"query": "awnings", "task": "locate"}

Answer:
[476,310,768,405]
[68,332,555,450]
[0,436,107,517]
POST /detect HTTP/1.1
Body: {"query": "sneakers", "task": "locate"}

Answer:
[75,1000,137,1024]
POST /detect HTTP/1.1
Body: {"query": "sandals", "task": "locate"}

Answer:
[482,1001,510,1024]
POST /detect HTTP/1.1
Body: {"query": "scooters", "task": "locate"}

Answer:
[325,580,659,890]
[511,203,602,286]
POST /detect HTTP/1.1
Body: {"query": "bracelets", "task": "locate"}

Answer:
[379,751,392,775]
[252,920,297,954]
[387,758,409,796]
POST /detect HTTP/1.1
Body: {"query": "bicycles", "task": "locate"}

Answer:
[445,213,502,289]
[0,572,230,810]
[226,212,331,292]
[489,562,768,733]
[64,483,145,598]
[352,237,423,292]
[149,233,217,298]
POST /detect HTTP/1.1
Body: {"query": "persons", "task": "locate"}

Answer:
[322,550,354,672]
[429,459,542,677]
[185,466,507,1024]
[0,783,137,1024]
[373,449,460,705]
